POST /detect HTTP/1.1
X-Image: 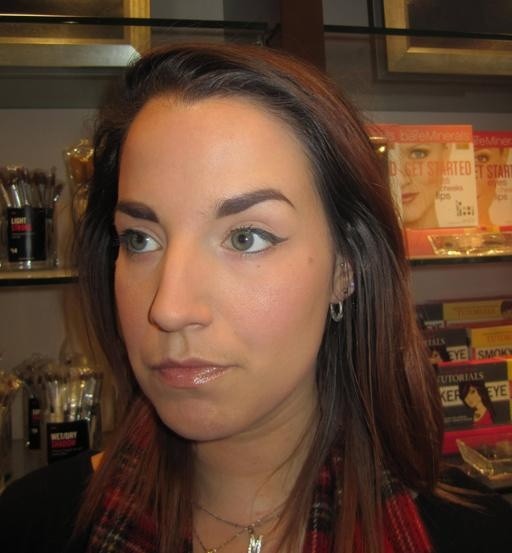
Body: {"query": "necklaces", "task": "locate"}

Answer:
[190,501,286,553]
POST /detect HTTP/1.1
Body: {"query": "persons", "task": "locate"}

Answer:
[475,148,509,226]
[1,42,512,553]
[400,142,451,229]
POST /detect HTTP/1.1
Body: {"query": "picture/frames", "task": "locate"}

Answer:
[371,0,512,81]
[1,1,152,108]
